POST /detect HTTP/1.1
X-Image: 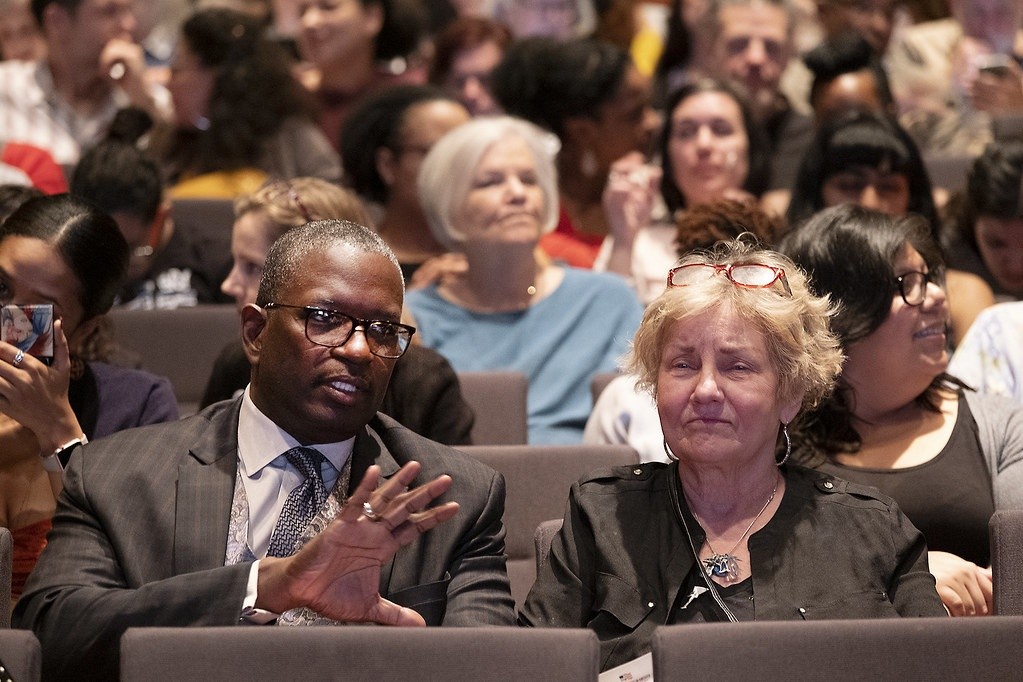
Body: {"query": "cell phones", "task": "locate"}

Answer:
[0,304,55,368]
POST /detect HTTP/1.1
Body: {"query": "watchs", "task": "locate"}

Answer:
[39,434,89,472]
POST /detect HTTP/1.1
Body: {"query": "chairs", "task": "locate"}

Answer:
[0,307,1023,682]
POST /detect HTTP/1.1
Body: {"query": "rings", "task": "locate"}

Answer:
[362,502,377,519]
[12,350,25,367]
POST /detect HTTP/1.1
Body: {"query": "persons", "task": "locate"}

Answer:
[776,201,1023,618]
[0,0,1023,463]
[196,176,475,447]
[10,218,517,682]
[515,232,950,682]
[0,192,179,592]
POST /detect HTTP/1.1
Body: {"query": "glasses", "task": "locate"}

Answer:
[262,304,416,359]
[254,174,312,225]
[665,265,794,299]
[891,270,938,305]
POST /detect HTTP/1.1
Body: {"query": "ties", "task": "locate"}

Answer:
[266,446,329,560]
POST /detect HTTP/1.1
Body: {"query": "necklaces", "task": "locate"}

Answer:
[682,482,778,582]
